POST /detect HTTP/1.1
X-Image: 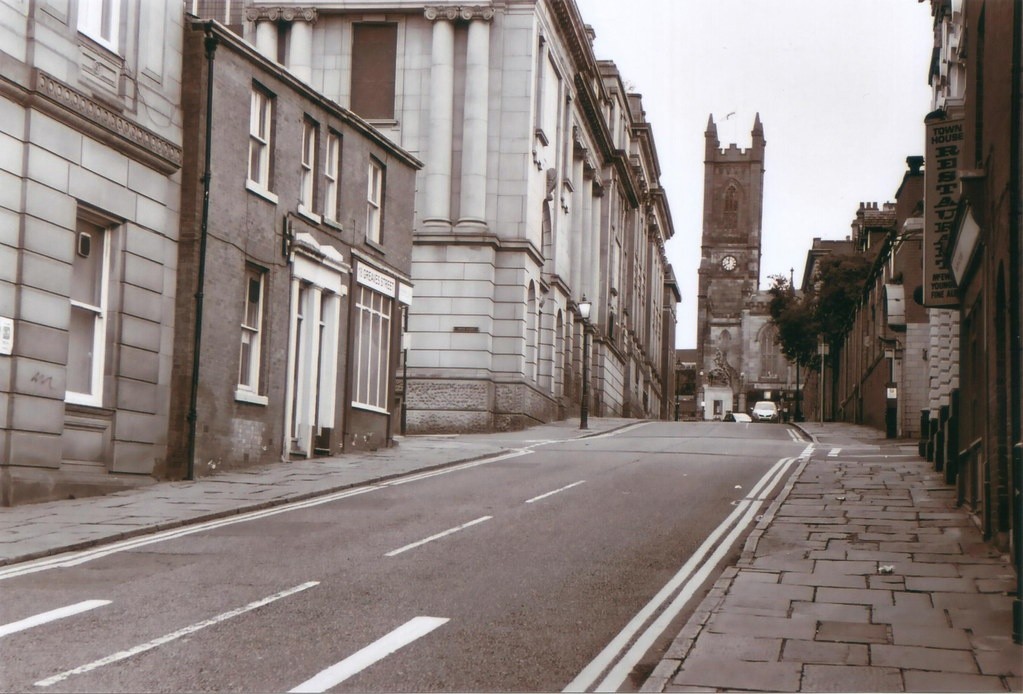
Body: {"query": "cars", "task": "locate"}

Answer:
[750,401,781,423]
[723,413,752,423]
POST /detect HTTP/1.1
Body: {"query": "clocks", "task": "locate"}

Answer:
[720,255,737,272]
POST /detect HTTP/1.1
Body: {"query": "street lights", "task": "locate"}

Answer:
[578,293,592,429]
[674,357,682,421]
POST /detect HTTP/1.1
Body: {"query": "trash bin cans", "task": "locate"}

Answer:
[783,402,791,420]
